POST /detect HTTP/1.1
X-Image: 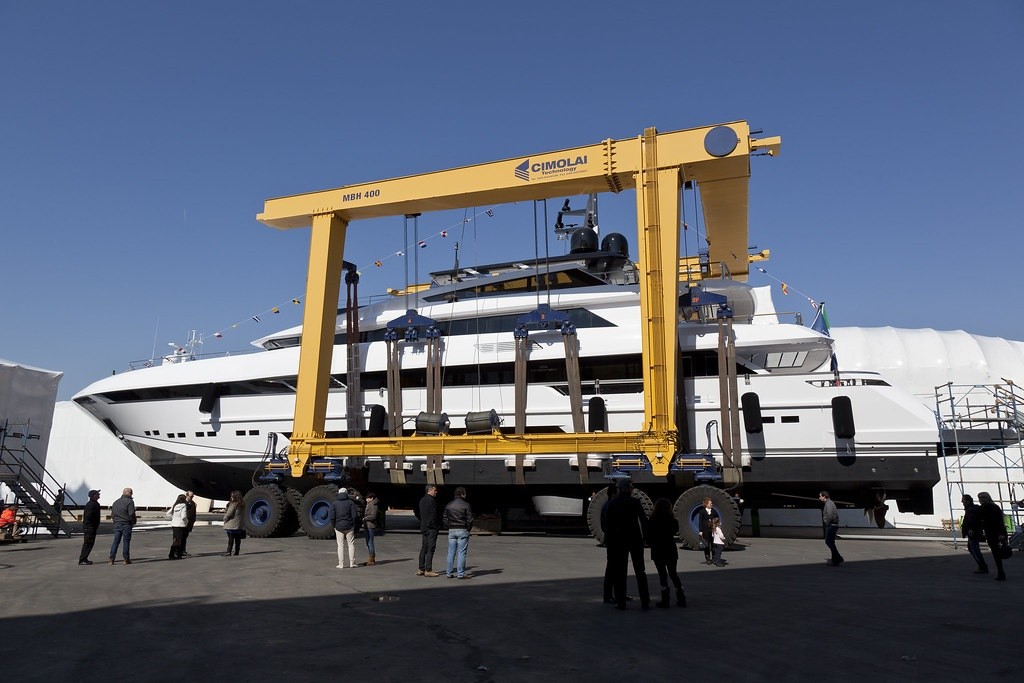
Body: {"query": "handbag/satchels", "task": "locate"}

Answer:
[1000,540,1012,559]
[232,530,246,539]
[693,536,709,551]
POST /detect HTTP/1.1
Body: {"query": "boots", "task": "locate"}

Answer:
[366,554,375,565]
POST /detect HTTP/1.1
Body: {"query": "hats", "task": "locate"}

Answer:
[338,487,348,494]
[9,505,18,511]
[89,490,101,497]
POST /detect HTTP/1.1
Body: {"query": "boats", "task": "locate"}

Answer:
[70,192,1024,515]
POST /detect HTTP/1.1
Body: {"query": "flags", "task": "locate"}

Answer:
[253,209,494,322]
[214,333,222,339]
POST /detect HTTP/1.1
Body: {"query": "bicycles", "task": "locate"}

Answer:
[0,516,30,537]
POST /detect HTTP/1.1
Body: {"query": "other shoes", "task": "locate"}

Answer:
[336,565,344,568]
[224,553,231,556]
[234,552,239,555]
[995,573,1006,580]
[828,558,844,566]
[974,567,988,574]
[350,564,358,568]
[416,569,471,579]
[705,558,728,567]
[109,560,114,565]
[79,560,93,565]
[169,552,192,560]
[603,592,686,611]
[124,560,131,564]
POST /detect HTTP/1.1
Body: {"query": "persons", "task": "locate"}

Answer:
[329,488,359,568]
[819,491,844,565]
[416,484,439,576]
[53,489,64,513]
[174,491,197,558]
[223,491,243,556]
[961,495,989,574]
[362,495,379,566]
[443,487,473,579]
[968,492,1007,581]
[644,499,687,608]
[165,494,189,559]
[600,484,633,602]
[109,488,137,565]
[606,480,650,609]
[78,490,101,565]
[712,518,725,567]
[698,497,720,564]
